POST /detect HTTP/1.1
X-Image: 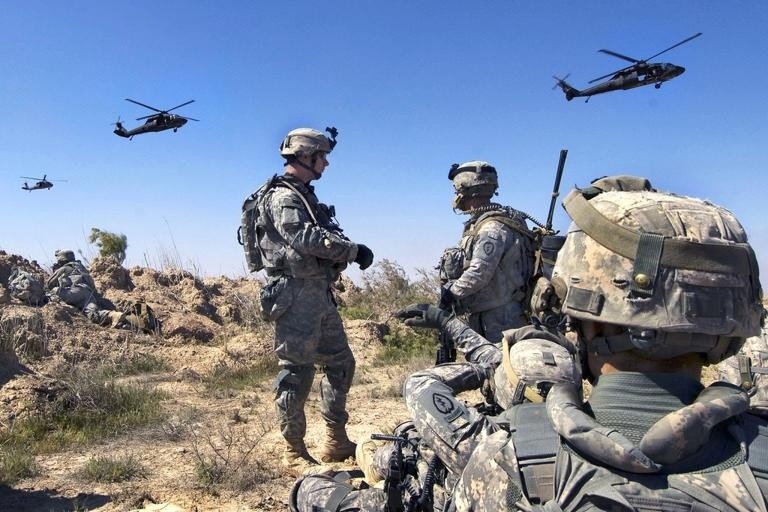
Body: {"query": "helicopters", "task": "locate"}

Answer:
[552,32,704,104]
[19,175,68,192]
[110,98,201,141]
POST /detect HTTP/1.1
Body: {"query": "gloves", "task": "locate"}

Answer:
[353,242,375,271]
[393,301,448,330]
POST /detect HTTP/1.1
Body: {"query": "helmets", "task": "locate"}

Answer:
[280,126,333,157]
[486,334,586,412]
[453,159,499,193]
[56,250,75,262]
[539,172,767,344]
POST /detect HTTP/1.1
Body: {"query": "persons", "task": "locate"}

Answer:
[437,160,567,365]
[237,126,375,474]
[288,175,768,512]
[47,249,126,328]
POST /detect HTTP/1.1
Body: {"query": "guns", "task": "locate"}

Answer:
[385,441,410,512]
[318,204,349,242]
[436,282,457,364]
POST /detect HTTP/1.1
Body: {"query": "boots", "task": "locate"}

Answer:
[321,417,356,463]
[281,436,320,472]
[109,310,125,328]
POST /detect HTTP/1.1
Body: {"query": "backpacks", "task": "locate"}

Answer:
[471,215,556,320]
[240,177,270,273]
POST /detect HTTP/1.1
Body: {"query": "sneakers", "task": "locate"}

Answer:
[354,440,385,485]
